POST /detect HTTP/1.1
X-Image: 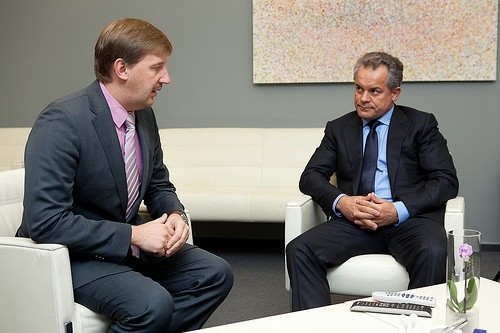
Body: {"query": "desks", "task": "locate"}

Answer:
[183,276,500,333]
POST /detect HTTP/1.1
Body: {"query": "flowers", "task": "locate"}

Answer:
[447,244,478,312]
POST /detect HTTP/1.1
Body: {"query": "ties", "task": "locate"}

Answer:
[359,120,381,200]
[123,114,140,257]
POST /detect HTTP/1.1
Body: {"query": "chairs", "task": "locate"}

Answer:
[285,173,465,296]
[0,167,193,333]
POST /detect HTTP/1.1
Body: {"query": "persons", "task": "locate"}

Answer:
[15,15,234,333]
[286,50,460,314]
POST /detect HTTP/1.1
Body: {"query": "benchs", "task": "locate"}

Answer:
[0,127,327,222]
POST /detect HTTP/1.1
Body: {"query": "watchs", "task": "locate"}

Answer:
[172,210,188,224]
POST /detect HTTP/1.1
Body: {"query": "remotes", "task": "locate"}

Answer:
[349,300,433,317]
[371,291,437,307]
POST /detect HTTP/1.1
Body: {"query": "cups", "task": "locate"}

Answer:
[446,229,481,333]
